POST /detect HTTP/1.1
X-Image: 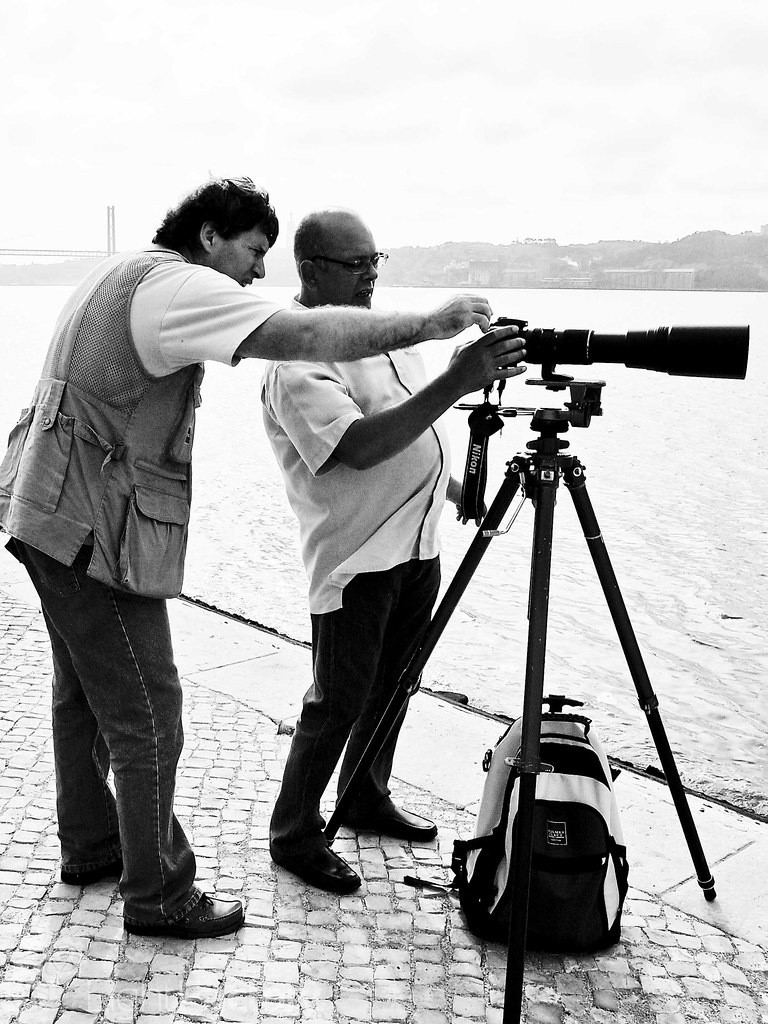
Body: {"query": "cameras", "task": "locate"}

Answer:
[492,317,750,380]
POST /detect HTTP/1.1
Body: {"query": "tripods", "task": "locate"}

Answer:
[320,377,716,1024]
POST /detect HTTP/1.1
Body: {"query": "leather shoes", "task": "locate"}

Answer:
[61,860,123,886]
[368,805,437,842]
[124,895,244,939]
[270,847,361,894]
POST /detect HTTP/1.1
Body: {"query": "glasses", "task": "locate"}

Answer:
[312,251,389,274]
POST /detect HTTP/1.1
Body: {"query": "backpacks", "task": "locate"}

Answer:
[404,712,629,955]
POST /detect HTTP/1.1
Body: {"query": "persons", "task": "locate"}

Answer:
[260,208,527,899]
[0,172,494,938]
